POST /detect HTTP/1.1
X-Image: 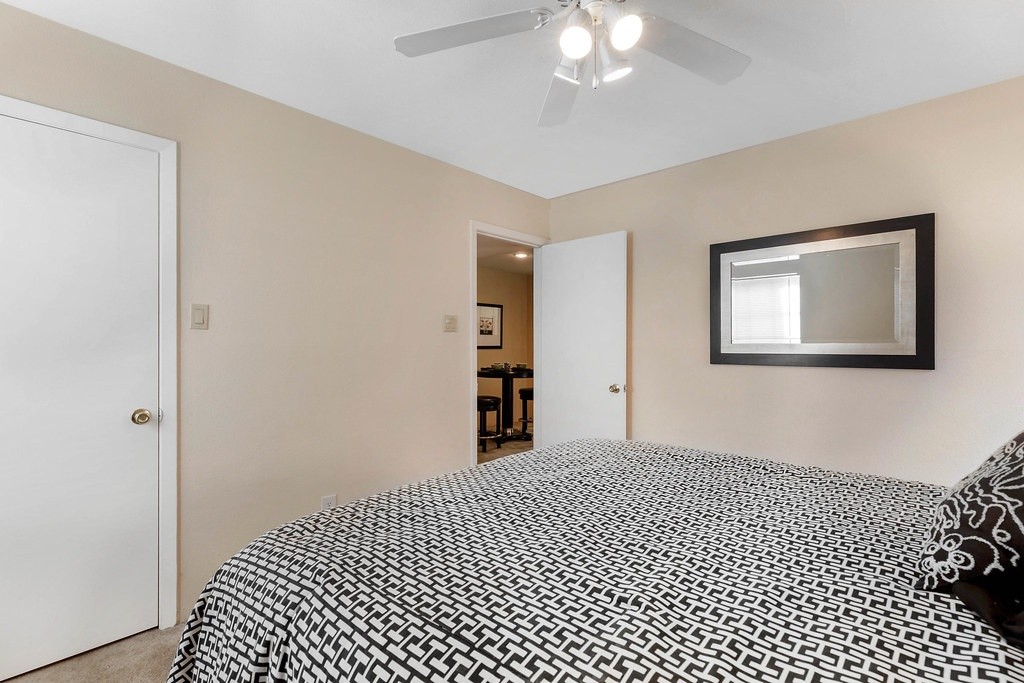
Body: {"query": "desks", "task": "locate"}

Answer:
[478,369,533,441]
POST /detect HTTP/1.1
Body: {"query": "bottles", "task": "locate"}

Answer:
[505,362,510,372]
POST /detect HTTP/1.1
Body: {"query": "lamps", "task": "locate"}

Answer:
[531,0,643,91]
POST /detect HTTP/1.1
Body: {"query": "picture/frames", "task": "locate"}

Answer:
[476,302,503,349]
[709,212,938,372]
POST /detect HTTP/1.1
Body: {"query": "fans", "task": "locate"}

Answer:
[394,0,752,130]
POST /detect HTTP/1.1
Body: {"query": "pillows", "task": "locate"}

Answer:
[909,430,1024,592]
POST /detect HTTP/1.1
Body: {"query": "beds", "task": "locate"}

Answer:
[169,439,1024,683]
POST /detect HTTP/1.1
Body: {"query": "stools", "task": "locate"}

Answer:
[518,388,533,442]
[477,396,502,453]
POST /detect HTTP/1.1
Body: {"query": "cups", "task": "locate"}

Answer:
[491,363,503,369]
[516,363,527,368]
